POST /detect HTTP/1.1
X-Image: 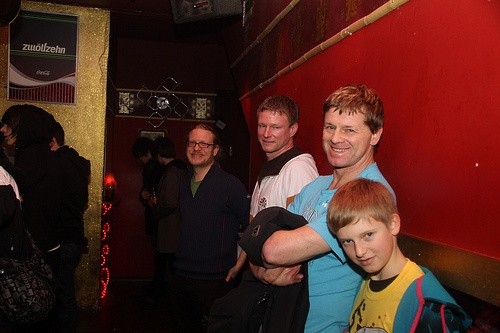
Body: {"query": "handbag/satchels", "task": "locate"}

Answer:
[0,250,57,325]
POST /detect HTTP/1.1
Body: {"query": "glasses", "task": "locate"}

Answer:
[186,140,215,148]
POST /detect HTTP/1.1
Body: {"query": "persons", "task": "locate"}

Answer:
[166,122,250,333]
[0,104,64,333]
[0,154,47,333]
[246,85,396,333]
[326,178,472,333]
[206,94,319,333]
[148,137,191,305]
[132,143,163,274]
[48,123,93,333]
